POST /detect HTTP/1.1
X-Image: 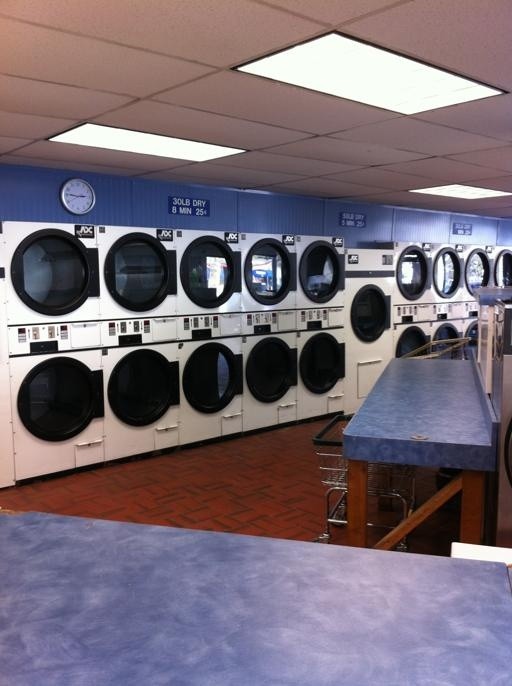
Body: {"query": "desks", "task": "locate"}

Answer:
[0,509,512,686]
[342,357,497,550]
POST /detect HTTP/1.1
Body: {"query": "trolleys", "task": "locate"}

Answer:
[398,336,473,362]
[311,409,420,553]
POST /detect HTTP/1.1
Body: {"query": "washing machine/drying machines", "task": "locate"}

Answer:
[0,221,105,488]
[358,237,512,365]
[177,230,244,448]
[344,247,393,416]
[241,230,297,435]
[97,223,180,461]
[295,234,345,422]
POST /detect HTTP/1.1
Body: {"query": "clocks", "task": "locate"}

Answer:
[60,179,96,215]
